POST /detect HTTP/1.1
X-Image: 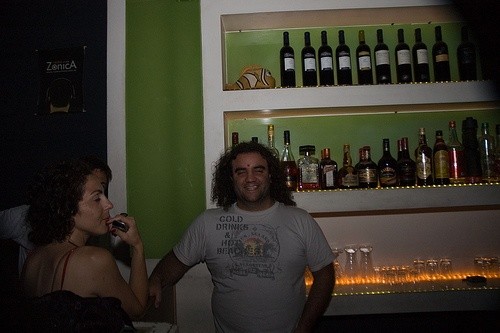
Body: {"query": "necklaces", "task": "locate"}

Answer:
[65,238,78,247]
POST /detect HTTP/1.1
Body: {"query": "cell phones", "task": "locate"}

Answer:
[112,220,129,232]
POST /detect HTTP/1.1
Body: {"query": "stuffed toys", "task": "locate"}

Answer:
[227,65,275,88]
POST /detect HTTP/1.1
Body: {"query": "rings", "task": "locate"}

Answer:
[120,213,128,217]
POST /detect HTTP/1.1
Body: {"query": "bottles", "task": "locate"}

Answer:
[378,139,398,188]
[355,146,378,188]
[280,32,296,88]
[231,125,297,191]
[318,31,334,86]
[395,29,412,84]
[336,30,352,86]
[414,128,433,186]
[449,117,500,178]
[397,137,416,186]
[412,28,430,83]
[457,26,477,81]
[337,145,358,189]
[432,26,451,82]
[374,29,391,84]
[297,145,337,190]
[356,30,373,84]
[301,32,317,87]
[433,130,450,185]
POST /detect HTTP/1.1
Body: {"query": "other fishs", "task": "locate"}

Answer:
[225,64,276,90]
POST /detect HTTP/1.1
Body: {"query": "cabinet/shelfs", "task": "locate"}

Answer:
[200,0,500,317]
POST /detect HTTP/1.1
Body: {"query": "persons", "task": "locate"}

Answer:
[12,155,149,333]
[0,151,131,293]
[138,141,336,333]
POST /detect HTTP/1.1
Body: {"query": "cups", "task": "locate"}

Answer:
[373,265,416,279]
[474,258,500,273]
[332,248,373,278]
[413,259,452,274]
